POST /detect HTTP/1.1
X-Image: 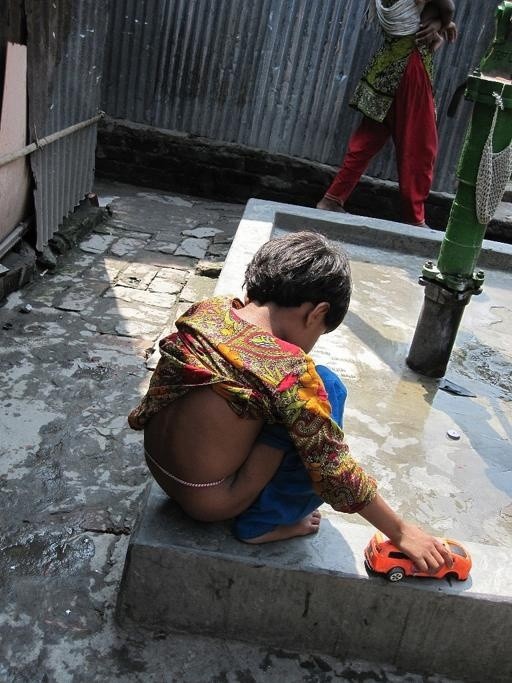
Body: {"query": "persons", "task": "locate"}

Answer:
[127,231,455,579]
[317,0,463,227]
